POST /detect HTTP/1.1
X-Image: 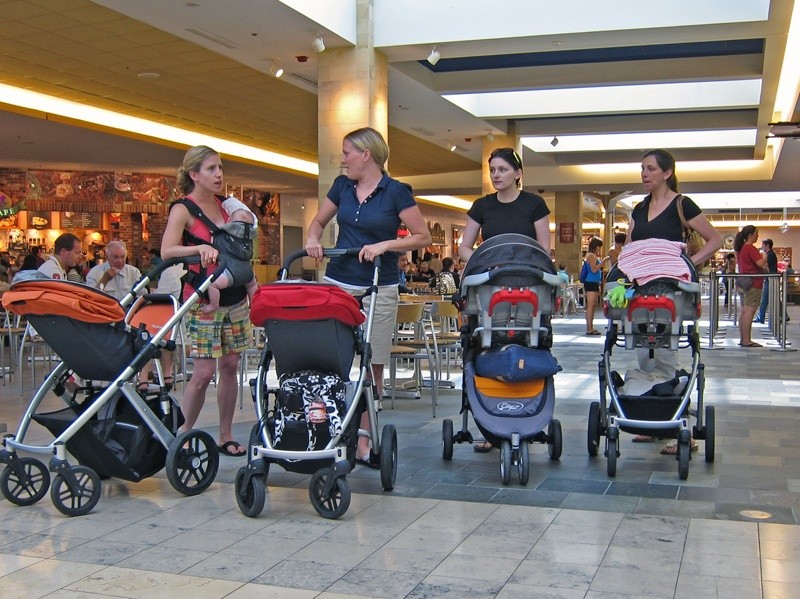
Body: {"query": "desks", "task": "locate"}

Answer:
[568,284,586,309]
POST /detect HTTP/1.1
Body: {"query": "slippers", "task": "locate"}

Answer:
[474,442,493,452]
[662,444,698,455]
[218,441,247,457]
[738,343,762,347]
[357,455,380,469]
[586,330,600,335]
[633,435,654,442]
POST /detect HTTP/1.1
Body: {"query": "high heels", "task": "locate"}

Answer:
[163,377,175,391]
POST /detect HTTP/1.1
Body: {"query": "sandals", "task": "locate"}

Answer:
[136,382,150,400]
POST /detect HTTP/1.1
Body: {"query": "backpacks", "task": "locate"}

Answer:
[580,254,597,283]
[274,370,346,462]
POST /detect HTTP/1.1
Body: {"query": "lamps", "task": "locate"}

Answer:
[447,142,456,152]
[551,135,558,147]
[269,59,284,79]
[311,32,326,53]
[427,46,440,66]
[486,130,495,142]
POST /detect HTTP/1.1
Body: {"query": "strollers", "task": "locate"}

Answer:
[442,232,564,484]
[235,248,398,517]
[0,253,229,516]
[586,237,715,479]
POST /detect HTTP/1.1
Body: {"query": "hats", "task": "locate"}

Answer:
[762,239,773,247]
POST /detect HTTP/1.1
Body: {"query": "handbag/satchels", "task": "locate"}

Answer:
[431,272,457,295]
[677,196,710,271]
[735,275,754,289]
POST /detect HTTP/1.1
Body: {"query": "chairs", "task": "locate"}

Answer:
[0,282,461,418]
[561,287,577,317]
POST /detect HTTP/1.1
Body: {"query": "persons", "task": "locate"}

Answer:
[201,209,257,317]
[458,147,553,453]
[617,150,725,456]
[159,145,253,456]
[0,224,793,348]
[579,237,610,336]
[304,127,432,467]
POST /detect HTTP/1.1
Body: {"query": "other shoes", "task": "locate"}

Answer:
[752,319,759,322]
[64,370,83,390]
[724,303,733,307]
[780,317,790,323]
